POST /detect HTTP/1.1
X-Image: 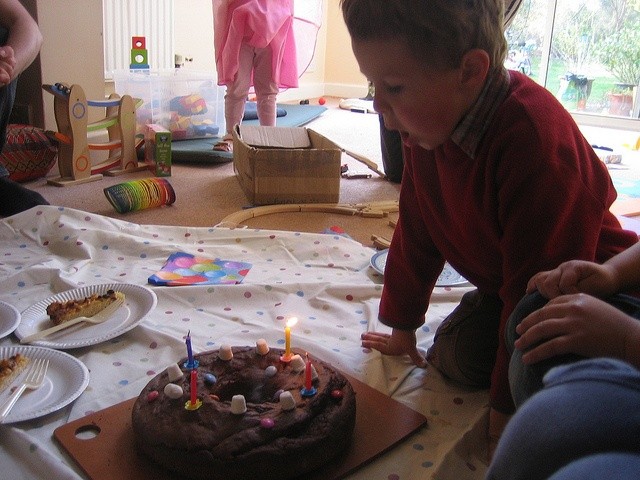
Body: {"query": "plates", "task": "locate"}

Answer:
[1,344,90,426]
[13,281,158,350]
[370,247,470,287]
[1,301,22,339]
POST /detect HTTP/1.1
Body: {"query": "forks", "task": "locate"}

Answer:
[1,357,50,423]
[20,297,126,344]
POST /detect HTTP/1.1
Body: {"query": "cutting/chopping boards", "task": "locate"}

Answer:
[54,348,428,479]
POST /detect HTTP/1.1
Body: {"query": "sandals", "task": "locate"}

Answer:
[213,134,234,152]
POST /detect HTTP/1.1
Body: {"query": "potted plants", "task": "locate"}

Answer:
[604,85,632,114]
[544,22,604,108]
[599,19,640,91]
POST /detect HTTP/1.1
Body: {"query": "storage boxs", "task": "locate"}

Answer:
[109,64,227,140]
[233,123,387,204]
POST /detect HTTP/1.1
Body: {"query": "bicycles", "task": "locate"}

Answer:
[503,40,531,77]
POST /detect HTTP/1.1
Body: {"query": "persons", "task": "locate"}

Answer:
[0,1,44,179]
[340,0,640,389]
[210,0,300,151]
[506,237,640,411]
[485,357,639,480]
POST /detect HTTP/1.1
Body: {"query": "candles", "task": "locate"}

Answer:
[303,352,313,388]
[184,330,194,362]
[283,317,296,357]
[189,364,197,404]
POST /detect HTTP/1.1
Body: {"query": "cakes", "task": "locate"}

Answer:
[46,289,126,325]
[0,353,29,392]
[132,327,356,474]
